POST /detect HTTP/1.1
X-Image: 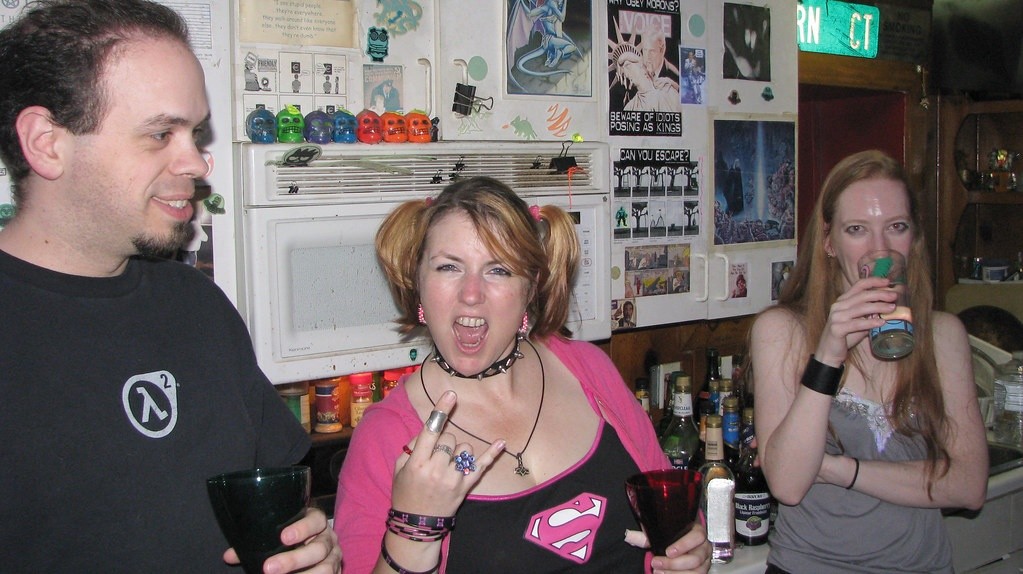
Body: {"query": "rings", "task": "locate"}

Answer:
[449,451,476,475]
[426,411,449,433]
[403,445,413,456]
[431,445,454,457]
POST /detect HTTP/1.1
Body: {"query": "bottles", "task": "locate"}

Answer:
[349,365,423,433]
[275,380,310,436]
[627,343,777,563]
[316,377,346,433]
[993,350,1023,449]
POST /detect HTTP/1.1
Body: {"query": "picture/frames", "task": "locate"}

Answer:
[706,104,797,251]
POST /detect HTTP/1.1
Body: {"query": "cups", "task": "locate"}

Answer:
[626,468,703,560]
[209,467,316,574]
[857,249,915,359]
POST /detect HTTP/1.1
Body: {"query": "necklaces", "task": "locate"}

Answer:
[430,332,524,380]
[421,336,545,476]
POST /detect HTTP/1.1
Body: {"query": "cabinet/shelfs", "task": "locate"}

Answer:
[705,450,1023,574]
[936,95,1023,326]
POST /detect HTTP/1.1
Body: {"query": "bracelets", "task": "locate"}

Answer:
[800,354,844,397]
[381,508,456,574]
[846,458,859,490]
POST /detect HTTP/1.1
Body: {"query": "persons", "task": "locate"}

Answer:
[743,150,990,574]
[0,1,343,574]
[731,275,747,298]
[611,301,635,327]
[681,50,706,104]
[615,27,680,105]
[625,250,690,298]
[607,16,681,112]
[334,177,713,574]
